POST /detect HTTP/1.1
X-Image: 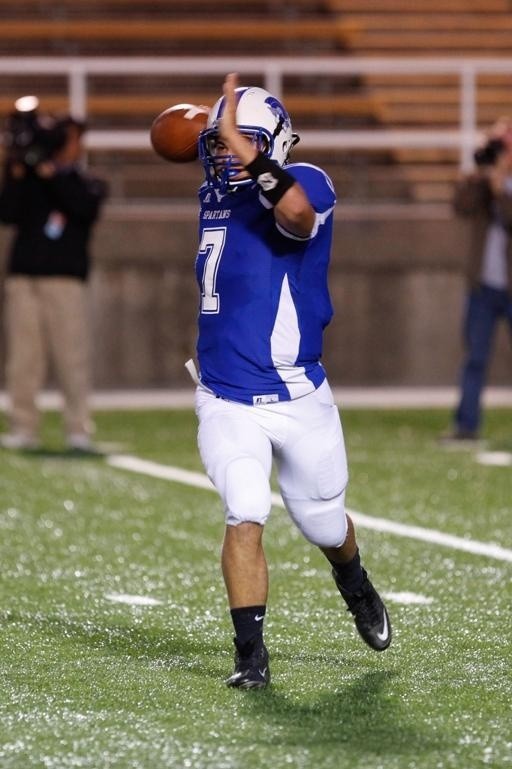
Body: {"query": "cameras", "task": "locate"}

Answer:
[474,139,503,165]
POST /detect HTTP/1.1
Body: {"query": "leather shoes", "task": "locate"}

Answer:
[330,568,390,652]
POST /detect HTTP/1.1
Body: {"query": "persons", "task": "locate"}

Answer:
[439,137,512,443]
[193,73,391,689]
[1,115,110,452]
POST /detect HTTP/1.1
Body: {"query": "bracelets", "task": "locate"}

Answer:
[246,151,297,208]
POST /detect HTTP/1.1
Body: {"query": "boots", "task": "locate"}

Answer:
[436,424,481,440]
[227,636,271,689]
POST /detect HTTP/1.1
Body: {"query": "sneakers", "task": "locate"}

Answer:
[3,425,93,452]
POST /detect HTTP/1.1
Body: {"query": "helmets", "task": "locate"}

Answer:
[199,86,299,192]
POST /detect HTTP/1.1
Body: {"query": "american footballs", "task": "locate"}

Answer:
[150,103,209,163]
